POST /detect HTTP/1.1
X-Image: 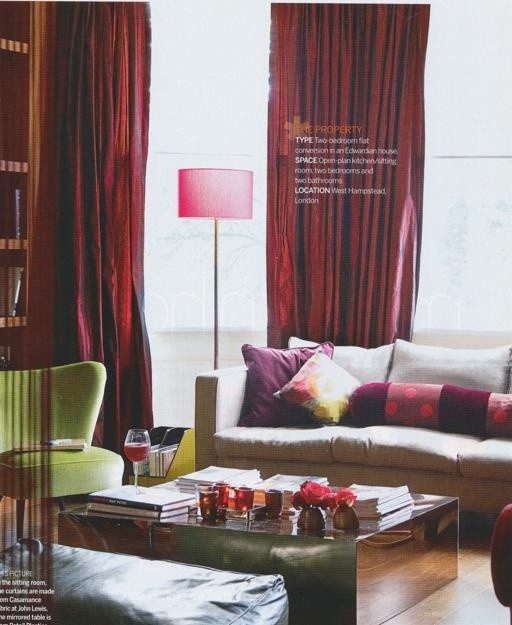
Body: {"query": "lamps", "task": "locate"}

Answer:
[176,169,255,371]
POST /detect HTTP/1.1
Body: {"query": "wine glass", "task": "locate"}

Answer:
[121,427,152,497]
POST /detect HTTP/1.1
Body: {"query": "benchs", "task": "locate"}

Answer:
[0,541,289,622]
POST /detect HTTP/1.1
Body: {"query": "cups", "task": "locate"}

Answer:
[195,480,282,523]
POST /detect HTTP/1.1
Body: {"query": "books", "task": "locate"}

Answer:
[13,438,86,452]
[82,444,413,529]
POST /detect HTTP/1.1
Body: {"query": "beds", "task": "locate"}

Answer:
[58,481,459,625]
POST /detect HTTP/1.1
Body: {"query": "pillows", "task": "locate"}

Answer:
[238,336,512,439]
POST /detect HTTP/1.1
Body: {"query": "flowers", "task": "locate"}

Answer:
[290,480,357,512]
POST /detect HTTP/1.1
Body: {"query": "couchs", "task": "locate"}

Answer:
[193,336,512,515]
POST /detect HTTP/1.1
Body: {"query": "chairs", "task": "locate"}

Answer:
[0,362,124,541]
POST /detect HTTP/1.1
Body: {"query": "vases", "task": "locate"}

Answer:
[297,506,359,530]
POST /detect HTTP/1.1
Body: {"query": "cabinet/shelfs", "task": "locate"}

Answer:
[0,2,59,373]
